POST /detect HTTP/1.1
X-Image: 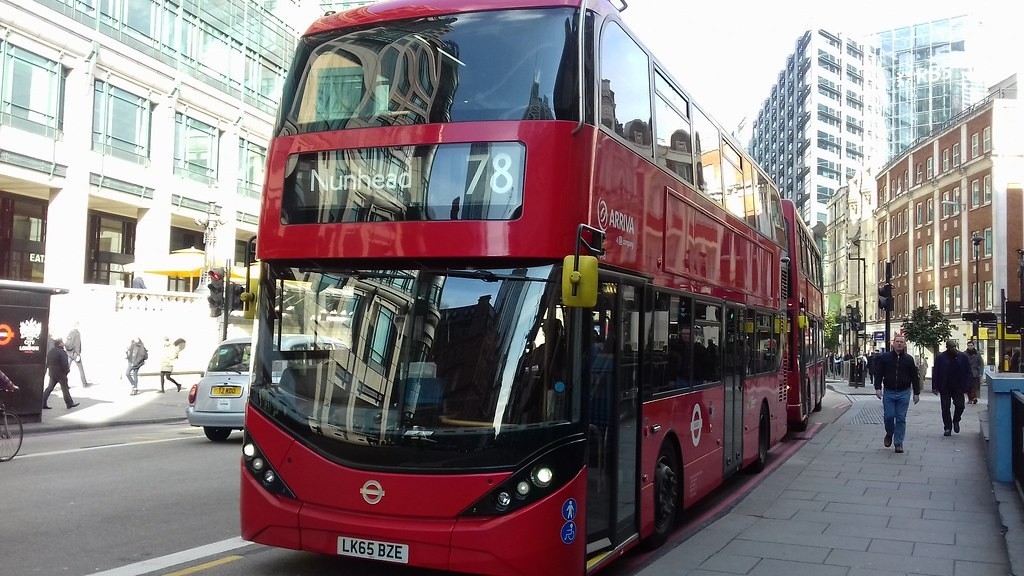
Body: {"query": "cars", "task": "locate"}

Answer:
[187,334,348,443]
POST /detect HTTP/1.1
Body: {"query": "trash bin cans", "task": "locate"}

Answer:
[985,371,1024,482]
[848,359,865,386]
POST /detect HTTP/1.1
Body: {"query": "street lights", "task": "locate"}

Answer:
[846,304,853,354]
[194,201,228,293]
[972,234,985,351]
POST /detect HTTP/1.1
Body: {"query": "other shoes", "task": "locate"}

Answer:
[83,383,92,388]
[967,399,978,404]
[944,420,961,436]
[131,389,139,395]
[43,406,51,409]
[157,390,165,393]
[67,402,80,409]
[177,384,182,392]
[884,434,905,453]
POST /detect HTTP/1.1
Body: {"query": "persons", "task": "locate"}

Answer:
[932,340,975,436]
[530,318,572,369]
[706,338,717,352]
[961,341,985,405]
[826,352,877,385]
[669,326,706,387]
[916,353,929,382]
[64,321,92,389]
[42,333,81,410]
[132,275,149,311]
[998,347,1021,373]
[125,336,149,396]
[0,370,20,394]
[873,335,921,453]
[156,336,182,394]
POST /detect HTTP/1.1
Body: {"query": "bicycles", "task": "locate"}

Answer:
[0,388,23,462]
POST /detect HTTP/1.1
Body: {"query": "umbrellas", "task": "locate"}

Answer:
[141,245,246,292]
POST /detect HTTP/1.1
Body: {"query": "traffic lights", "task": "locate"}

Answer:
[878,284,894,311]
[852,314,862,330]
[726,311,734,321]
[228,284,244,311]
[208,268,224,318]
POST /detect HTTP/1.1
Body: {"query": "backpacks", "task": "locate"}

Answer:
[65,336,74,351]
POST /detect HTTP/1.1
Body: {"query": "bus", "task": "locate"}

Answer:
[238,1,790,576]
[782,199,827,434]
